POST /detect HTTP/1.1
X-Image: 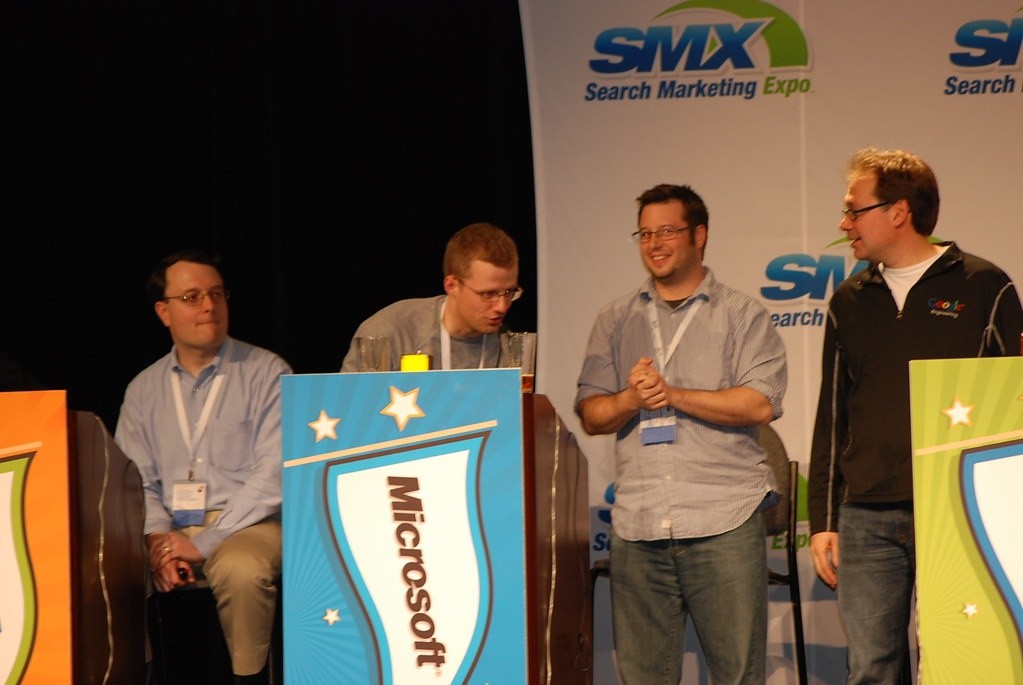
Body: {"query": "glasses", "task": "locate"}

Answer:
[631,225,694,245]
[165,289,230,307]
[458,281,523,303]
[841,201,887,220]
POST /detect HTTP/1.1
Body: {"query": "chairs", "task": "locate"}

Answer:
[591,425,808,685]
[145,577,278,685]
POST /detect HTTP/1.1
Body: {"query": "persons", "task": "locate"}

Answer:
[806,145,1022,685]
[573,184,788,685]
[339,223,523,372]
[112,254,294,685]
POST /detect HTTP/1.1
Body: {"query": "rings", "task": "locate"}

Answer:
[164,547,171,553]
